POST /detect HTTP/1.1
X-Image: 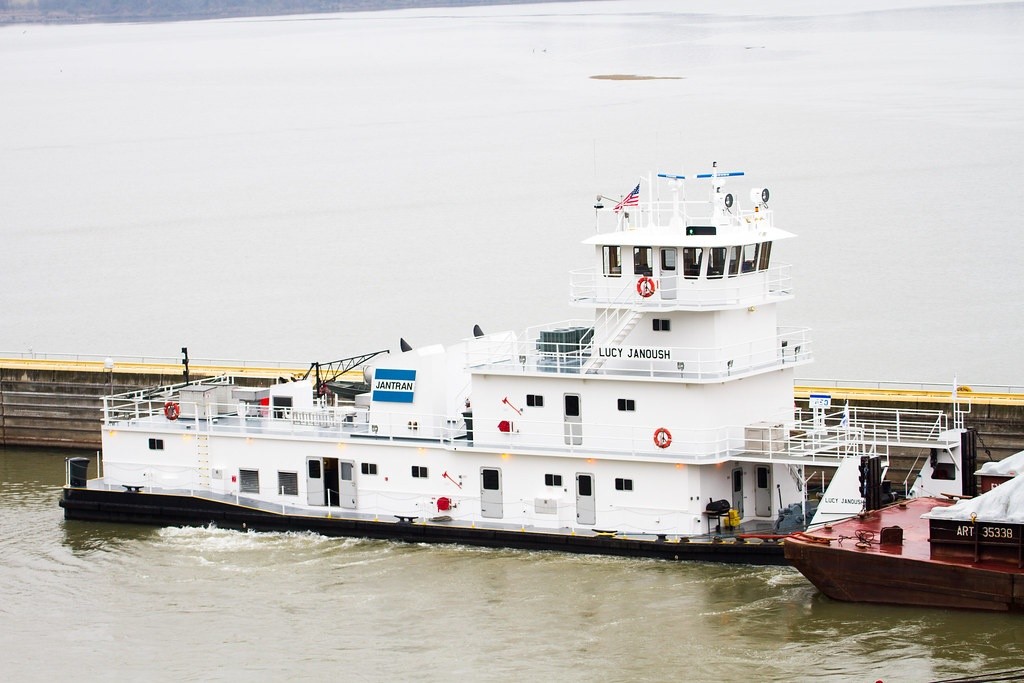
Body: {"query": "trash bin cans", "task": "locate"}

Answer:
[70,457,90,487]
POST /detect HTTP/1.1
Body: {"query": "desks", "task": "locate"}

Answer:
[703,507,736,534]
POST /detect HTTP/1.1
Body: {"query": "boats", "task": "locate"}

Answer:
[59,163,976,563]
[784,451,1024,612]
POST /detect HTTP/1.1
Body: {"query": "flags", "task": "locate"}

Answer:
[840,405,845,428]
[613,183,640,214]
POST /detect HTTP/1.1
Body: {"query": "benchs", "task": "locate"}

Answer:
[592,529,617,537]
[122,485,145,492]
[394,515,418,523]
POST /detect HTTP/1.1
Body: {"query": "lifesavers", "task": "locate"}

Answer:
[637,276,655,297]
[653,426,672,448]
[164,401,180,420]
